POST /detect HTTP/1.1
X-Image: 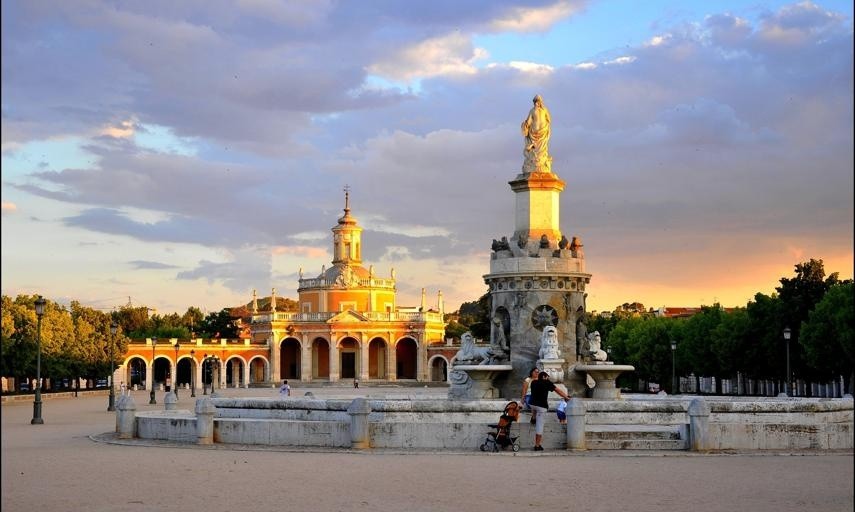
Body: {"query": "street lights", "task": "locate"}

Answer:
[606,345,612,360]
[190,349,197,396]
[29,296,47,424]
[106,322,117,411]
[150,335,158,405]
[203,353,221,395]
[671,340,677,393]
[174,342,179,400]
[782,326,792,394]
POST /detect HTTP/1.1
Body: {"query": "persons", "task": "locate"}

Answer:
[520,367,539,409]
[557,395,571,425]
[528,370,571,451]
[119,380,125,396]
[353,378,359,387]
[521,93,553,173]
[126,381,130,395]
[279,379,291,399]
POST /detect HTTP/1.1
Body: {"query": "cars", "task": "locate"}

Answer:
[20,383,30,393]
[63,378,68,388]
[96,379,108,390]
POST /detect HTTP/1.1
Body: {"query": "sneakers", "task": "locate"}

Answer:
[534,445,544,451]
[530,417,536,424]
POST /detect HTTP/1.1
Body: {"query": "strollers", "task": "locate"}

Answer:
[480,400,522,453]
